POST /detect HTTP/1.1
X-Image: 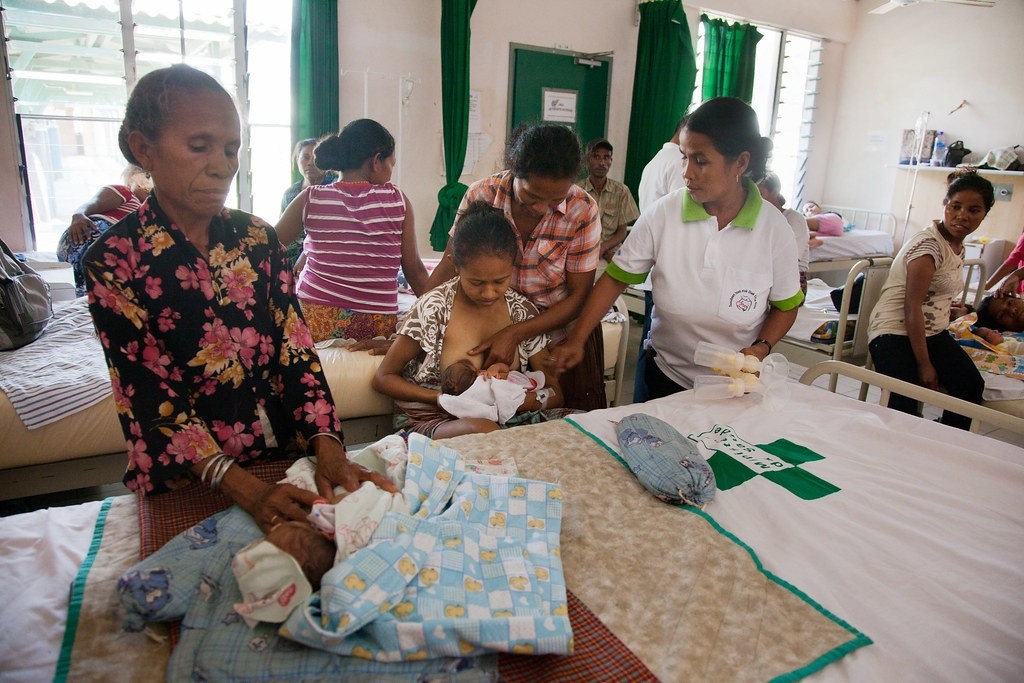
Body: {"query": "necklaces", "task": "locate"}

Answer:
[341,180,369,184]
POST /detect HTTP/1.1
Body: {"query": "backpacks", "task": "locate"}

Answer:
[0,238,55,352]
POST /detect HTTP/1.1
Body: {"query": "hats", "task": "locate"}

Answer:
[586,137,613,156]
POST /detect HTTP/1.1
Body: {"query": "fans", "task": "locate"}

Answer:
[869,0,996,16]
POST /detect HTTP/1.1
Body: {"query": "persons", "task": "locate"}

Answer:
[866,165,995,432]
[755,170,810,307]
[56,183,151,299]
[971,328,1024,356]
[974,266,1024,333]
[576,138,641,263]
[345,123,608,412]
[984,225,1024,294]
[803,201,843,249]
[274,118,429,341]
[278,138,339,260]
[632,112,693,404]
[441,363,546,396]
[370,199,588,441]
[545,95,805,402]
[268,520,336,593]
[84,65,400,537]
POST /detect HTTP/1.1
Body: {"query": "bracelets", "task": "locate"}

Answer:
[751,338,772,356]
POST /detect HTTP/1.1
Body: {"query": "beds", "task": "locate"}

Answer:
[0,360,1024,683]
[832,258,1024,434]
[795,205,895,273]
[0,252,630,519]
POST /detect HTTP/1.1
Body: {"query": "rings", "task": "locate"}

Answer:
[546,356,558,362]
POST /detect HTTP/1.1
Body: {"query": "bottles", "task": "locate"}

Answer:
[693,340,764,400]
[933,131,946,162]
